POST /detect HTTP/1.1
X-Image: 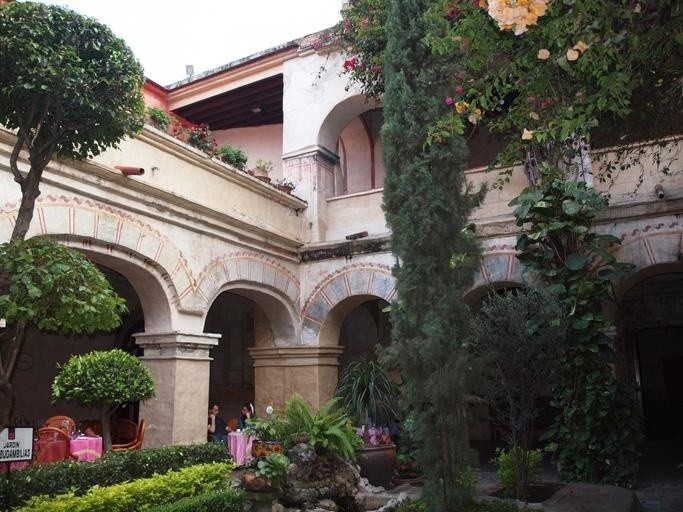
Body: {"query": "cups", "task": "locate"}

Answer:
[236,429,240,432]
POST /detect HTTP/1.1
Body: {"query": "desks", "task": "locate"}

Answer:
[222,429,259,462]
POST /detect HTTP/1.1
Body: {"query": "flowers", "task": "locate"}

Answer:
[274,177,295,187]
[251,161,273,171]
[179,122,219,154]
[352,425,393,446]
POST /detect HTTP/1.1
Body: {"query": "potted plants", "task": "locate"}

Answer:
[246,416,286,459]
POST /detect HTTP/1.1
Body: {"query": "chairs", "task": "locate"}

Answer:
[27,414,146,470]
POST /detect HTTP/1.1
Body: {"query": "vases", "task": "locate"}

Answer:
[276,184,293,195]
[252,170,270,183]
[352,447,399,489]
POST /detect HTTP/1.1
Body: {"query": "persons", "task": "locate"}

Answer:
[208,404,231,441]
[238,402,256,431]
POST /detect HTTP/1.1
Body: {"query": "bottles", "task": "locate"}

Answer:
[70,422,83,440]
